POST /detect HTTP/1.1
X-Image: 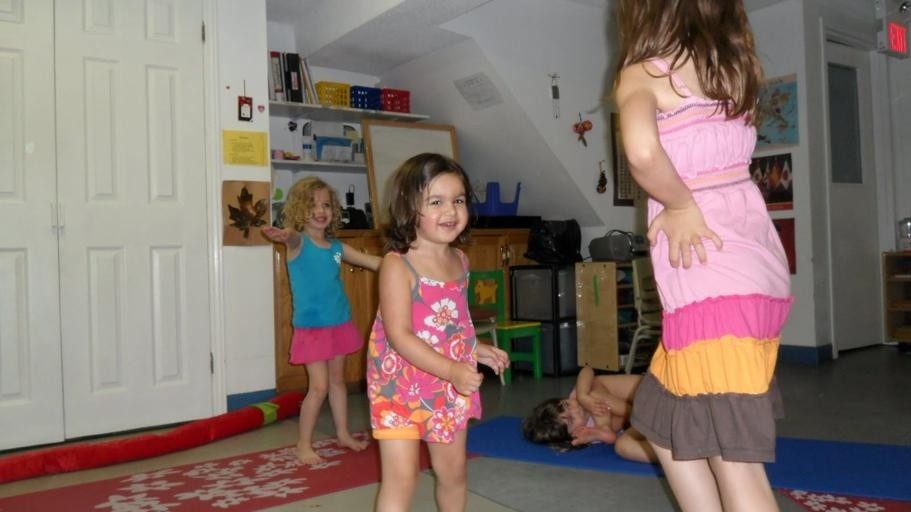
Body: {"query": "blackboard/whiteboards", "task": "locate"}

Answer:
[363,119,459,231]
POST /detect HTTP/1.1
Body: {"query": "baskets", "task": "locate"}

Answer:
[379,86,412,114]
[311,78,351,109]
[348,83,381,111]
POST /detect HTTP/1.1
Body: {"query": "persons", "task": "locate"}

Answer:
[587,0,793,511]
[523,363,657,464]
[364,153,511,511]
[262,177,383,465]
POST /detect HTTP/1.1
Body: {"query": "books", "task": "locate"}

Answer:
[267,49,321,105]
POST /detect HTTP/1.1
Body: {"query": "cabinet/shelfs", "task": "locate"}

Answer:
[269,99,431,167]
[575,260,657,373]
[882,250,911,344]
[457,228,540,344]
[273,229,385,394]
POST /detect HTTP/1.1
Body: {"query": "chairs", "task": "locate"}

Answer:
[625,258,664,376]
[468,266,544,386]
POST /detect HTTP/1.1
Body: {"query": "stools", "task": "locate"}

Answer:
[468,182,521,218]
[467,309,505,387]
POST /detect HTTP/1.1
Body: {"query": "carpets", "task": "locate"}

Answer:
[778,489,911,511]
[468,415,911,502]
[0,425,477,511]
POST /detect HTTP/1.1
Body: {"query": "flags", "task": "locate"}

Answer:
[750,158,792,200]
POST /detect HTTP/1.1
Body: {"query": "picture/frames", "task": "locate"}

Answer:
[611,111,635,207]
